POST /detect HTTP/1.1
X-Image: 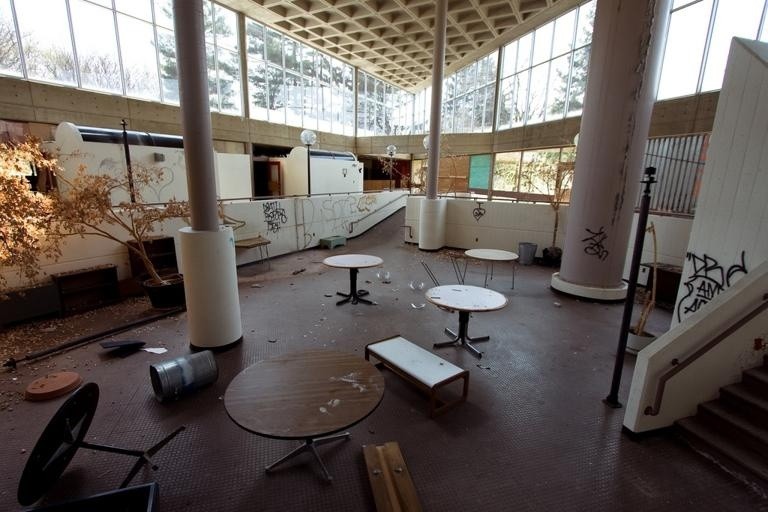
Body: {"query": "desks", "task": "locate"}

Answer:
[462,248,518,290]
[17,382,185,508]
[423,284,508,357]
[235,235,272,272]
[222,348,387,481]
[322,254,384,306]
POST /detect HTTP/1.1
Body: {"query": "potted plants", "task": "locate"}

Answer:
[518,126,578,270]
[0,130,226,312]
[620,220,658,356]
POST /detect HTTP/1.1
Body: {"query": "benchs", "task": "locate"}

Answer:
[364,335,471,415]
[320,235,347,250]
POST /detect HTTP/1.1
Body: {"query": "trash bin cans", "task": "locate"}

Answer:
[518,242,537,265]
[149,350,219,404]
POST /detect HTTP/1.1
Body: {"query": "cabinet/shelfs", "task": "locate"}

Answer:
[639,262,684,314]
[50,234,179,318]
